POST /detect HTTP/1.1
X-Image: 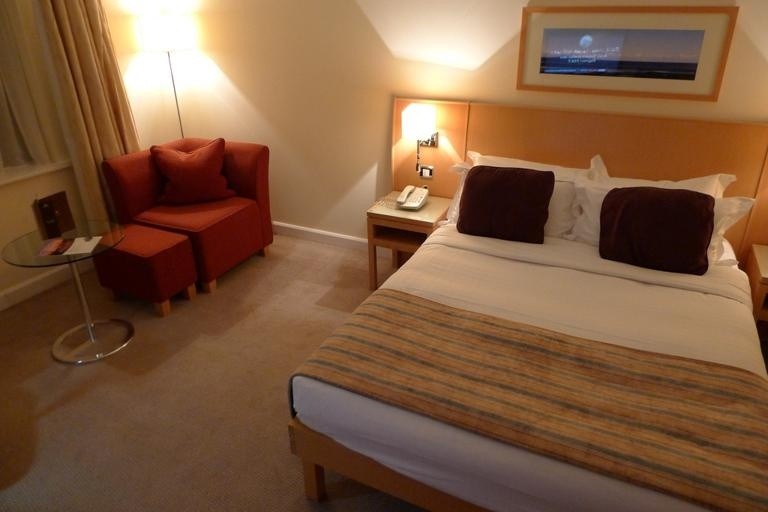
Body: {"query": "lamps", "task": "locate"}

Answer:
[401,103,439,148]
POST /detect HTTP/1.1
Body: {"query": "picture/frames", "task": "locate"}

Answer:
[516,4,739,102]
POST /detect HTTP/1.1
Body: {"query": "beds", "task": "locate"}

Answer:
[287,95,768,512]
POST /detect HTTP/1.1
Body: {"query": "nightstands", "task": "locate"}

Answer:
[366,190,453,291]
[746,244,768,322]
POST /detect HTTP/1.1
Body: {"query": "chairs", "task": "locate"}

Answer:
[101,137,274,293]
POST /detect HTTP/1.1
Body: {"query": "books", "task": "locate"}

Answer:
[33,235,104,257]
[32,190,76,241]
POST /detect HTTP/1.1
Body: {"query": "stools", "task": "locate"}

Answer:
[94,221,199,317]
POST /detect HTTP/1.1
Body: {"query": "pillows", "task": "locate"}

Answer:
[563,171,756,266]
[465,146,589,180]
[456,165,555,244]
[437,161,583,241]
[599,186,715,275]
[149,137,237,203]
[589,152,737,197]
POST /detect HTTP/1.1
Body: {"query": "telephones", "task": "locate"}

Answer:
[395,184,429,210]
